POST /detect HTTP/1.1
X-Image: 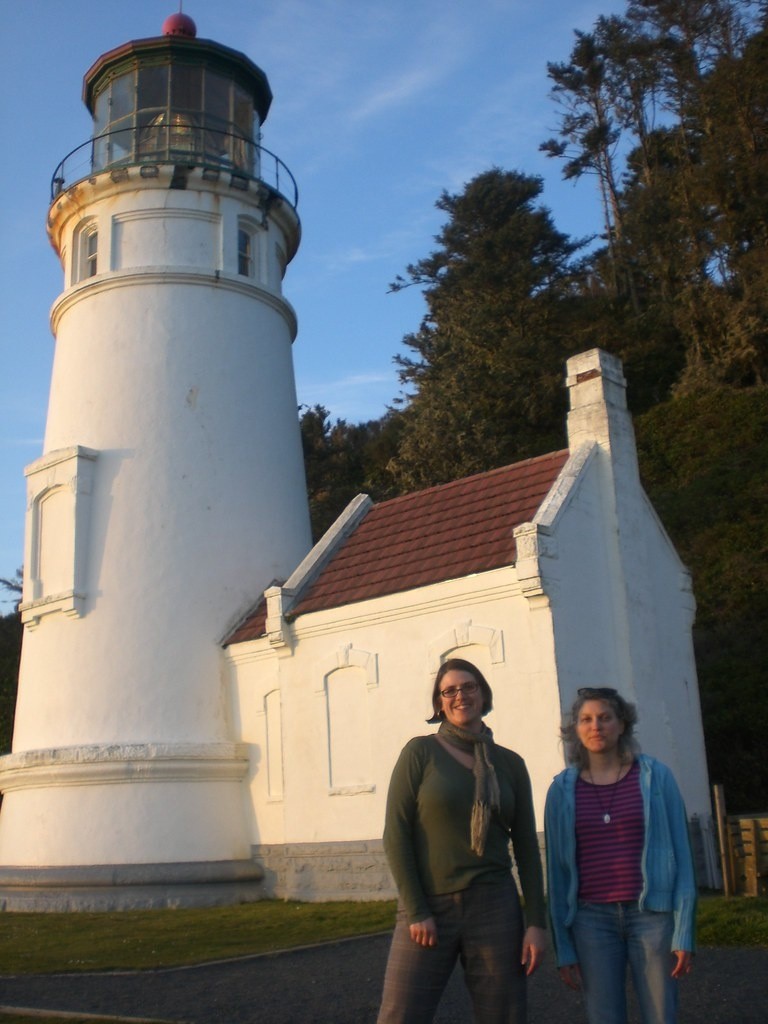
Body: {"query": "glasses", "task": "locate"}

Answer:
[440,683,480,698]
[577,687,627,726]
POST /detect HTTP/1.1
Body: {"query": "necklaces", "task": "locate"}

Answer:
[586,759,623,823]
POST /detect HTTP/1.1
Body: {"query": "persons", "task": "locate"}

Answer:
[376,658,546,1024]
[543,687,697,1024]
